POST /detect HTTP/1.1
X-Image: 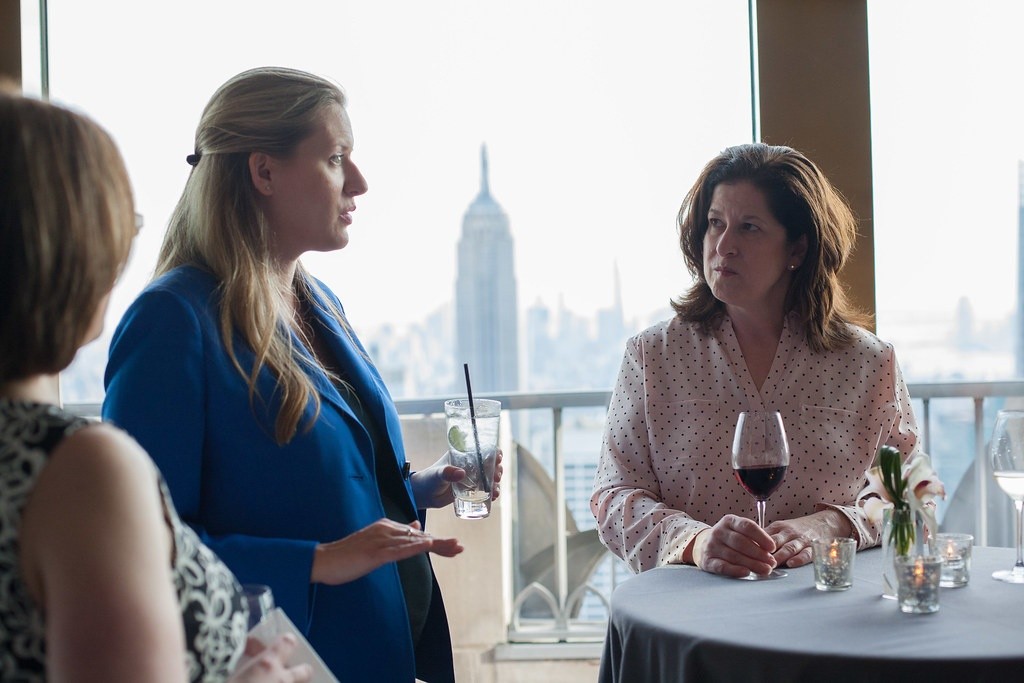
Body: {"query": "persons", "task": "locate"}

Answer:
[590,143,923,577]
[101,67,504,683]
[0,91,312,683]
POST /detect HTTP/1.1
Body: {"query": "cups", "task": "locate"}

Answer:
[811,536,858,592]
[228,584,275,656]
[445,399,501,519]
[926,533,975,588]
[893,554,944,614]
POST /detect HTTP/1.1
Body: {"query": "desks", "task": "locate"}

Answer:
[597,544,1024,683]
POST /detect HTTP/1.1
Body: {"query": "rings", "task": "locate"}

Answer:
[407,529,412,535]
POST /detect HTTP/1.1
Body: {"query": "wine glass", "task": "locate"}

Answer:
[988,410,1024,584]
[734,411,789,582]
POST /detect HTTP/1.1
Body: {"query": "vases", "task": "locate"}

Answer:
[883,507,923,601]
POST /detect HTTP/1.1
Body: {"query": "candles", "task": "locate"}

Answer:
[893,555,942,613]
[809,537,857,591]
[928,533,974,588]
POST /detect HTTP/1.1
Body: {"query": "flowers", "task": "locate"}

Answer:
[875,444,947,553]
